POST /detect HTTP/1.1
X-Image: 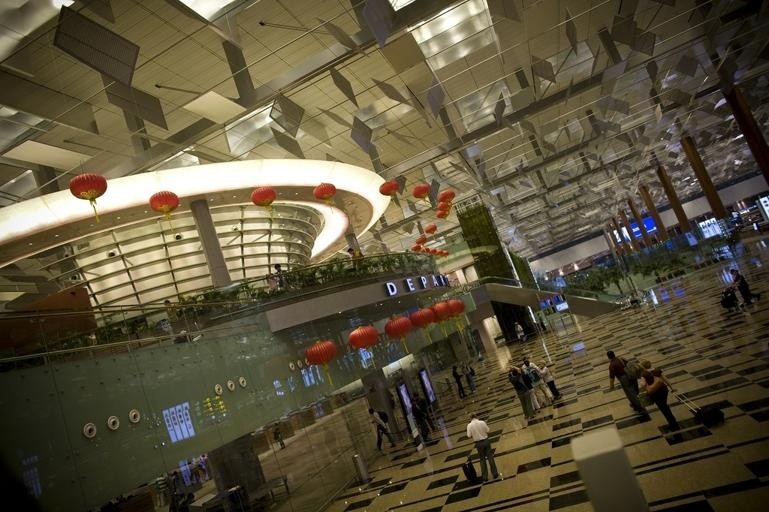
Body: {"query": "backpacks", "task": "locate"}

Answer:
[462,367,475,377]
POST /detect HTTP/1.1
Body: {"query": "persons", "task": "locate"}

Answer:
[153,454,212,511]
[387,389,397,409]
[630,292,641,309]
[275,428,286,449]
[730,268,760,305]
[607,351,676,430]
[411,392,438,443]
[453,360,477,400]
[467,412,499,482]
[514,312,547,344]
[509,357,562,419]
[155,248,435,335]
[369,408,396,451]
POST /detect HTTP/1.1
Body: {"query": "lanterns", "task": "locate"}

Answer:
[313,183,336,214]
[305,298,465,366]
[379,180,455,258]
[69,174,108,222]
[250,187,275,216]
[149,191,179,232]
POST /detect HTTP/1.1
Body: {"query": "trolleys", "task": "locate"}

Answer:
[719,286,741,313]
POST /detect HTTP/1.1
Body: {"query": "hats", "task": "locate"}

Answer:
[537,359,546,365]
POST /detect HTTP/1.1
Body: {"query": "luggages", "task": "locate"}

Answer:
[671,389,724,428]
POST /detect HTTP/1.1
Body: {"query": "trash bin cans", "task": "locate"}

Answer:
[352,453,371,484]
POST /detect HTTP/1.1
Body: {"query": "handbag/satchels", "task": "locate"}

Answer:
[638,391,655,407]
[462,461,477,482]
[624,361,644,378]
[377,411,388,423]
